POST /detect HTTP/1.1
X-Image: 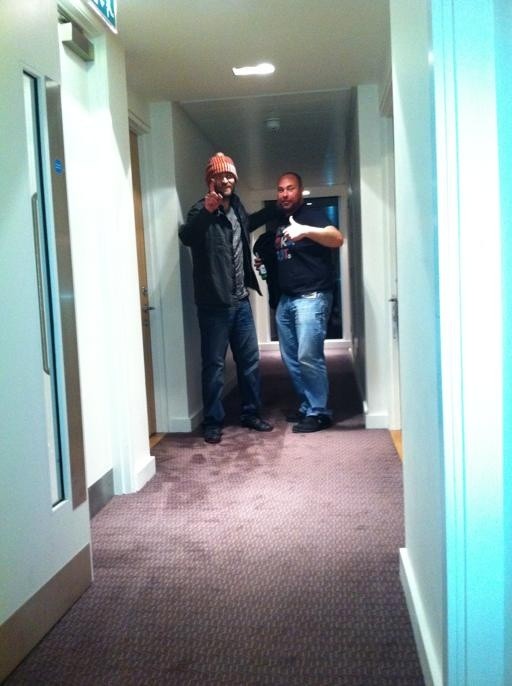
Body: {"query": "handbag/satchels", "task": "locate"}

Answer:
[252,232,278,277]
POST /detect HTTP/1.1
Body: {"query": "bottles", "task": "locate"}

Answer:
[254,252,269,281]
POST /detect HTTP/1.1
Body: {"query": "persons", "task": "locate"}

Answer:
[254,172,343,432]
[179,152,279,444]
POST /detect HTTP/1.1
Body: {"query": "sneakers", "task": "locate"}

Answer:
[205,424,221,443]
[293,414,332,431]
[286,410,304,421]
[241,415,272,431]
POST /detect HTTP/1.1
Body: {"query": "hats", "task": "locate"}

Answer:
[206,156,238,184]
